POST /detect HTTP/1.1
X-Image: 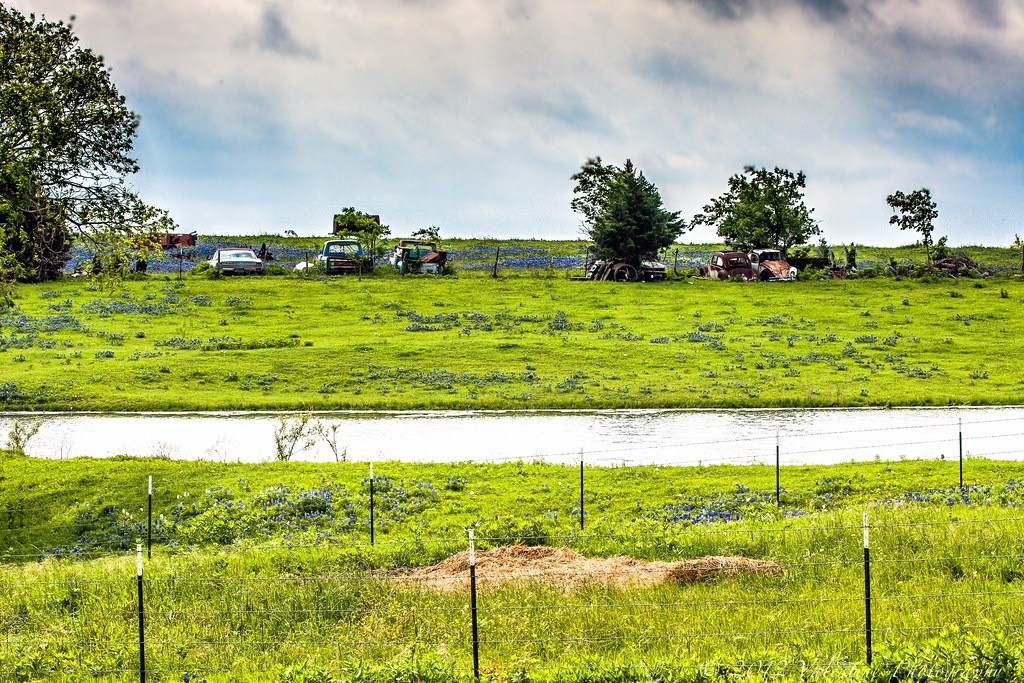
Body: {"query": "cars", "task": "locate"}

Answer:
[211,247,264,276]
[748,247,797,282]
[700,251,759,283]
[642,249,667,282]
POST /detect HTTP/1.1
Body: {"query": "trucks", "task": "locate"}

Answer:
[395,240,449,275]
[320,240,375,273]
